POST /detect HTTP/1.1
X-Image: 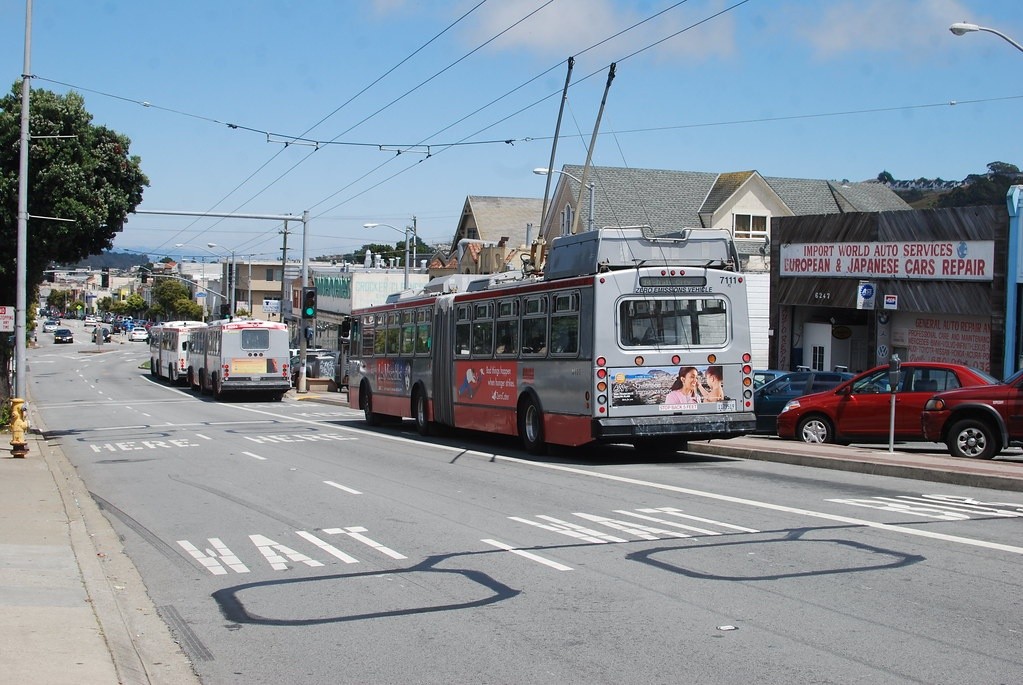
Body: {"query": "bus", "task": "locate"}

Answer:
[182,316,292,401]
[340,225,756,459]
[146,321,207,385]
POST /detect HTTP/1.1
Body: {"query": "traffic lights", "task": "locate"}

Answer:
[302,287,317,318]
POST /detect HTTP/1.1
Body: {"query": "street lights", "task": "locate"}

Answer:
[175,244,230,304]
[207,242,236,317]
[364,223,410,289]
[133,266,151,308]
[182,259,205,321]
[533,168,594,231]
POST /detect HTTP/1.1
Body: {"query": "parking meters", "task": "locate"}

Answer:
[888,354,902,452]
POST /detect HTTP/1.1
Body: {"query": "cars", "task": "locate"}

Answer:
[103,312,156,342]
[753,368,901,435]
[43,316,73,344]
[777,362,1000,446]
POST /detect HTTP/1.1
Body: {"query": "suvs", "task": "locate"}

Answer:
[921,368,1023,460]
[289,349,331,377]
[92,327,111,343]
[84,316,96,327]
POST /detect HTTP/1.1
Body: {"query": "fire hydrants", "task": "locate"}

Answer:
[9,399,31,459]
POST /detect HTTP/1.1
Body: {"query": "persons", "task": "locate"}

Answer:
[665,367,701,404]
[696,366,723,402]
